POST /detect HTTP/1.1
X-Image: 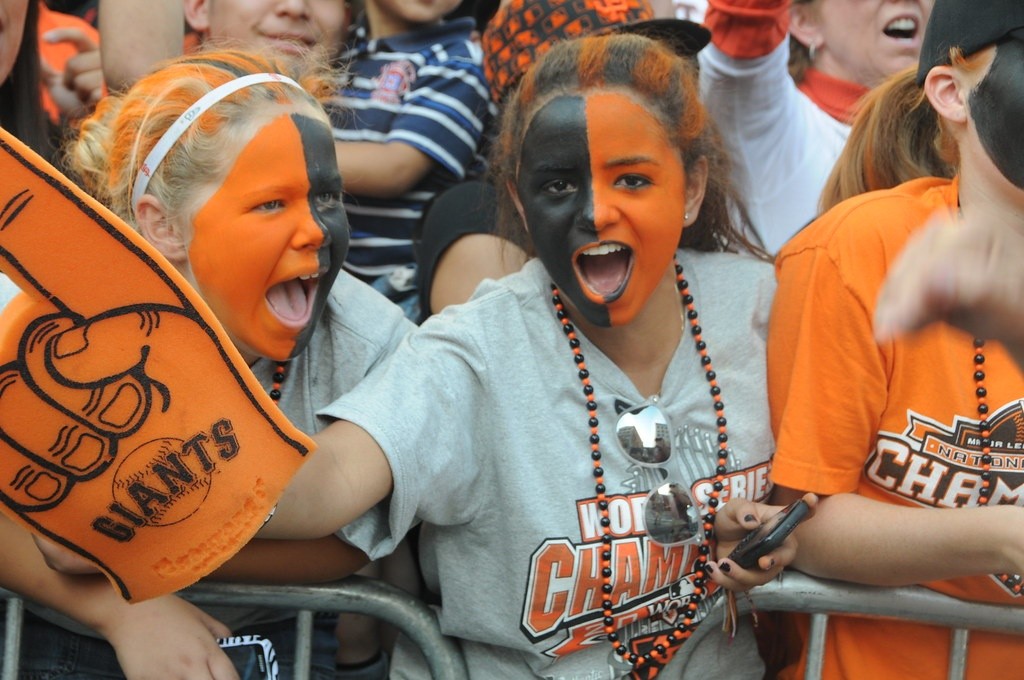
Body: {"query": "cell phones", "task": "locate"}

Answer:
[727,499,810,570]
[215,635,279,680]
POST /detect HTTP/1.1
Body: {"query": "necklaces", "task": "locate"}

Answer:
[551,252,729,664]
[970,337,1024,595]
[268,361,286,407]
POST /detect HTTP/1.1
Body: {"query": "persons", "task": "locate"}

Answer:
[0,0,1024,680]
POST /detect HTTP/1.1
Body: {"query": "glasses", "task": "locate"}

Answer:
[614,394,706,548]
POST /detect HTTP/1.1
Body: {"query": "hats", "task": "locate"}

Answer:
[914,0,1023,87]
